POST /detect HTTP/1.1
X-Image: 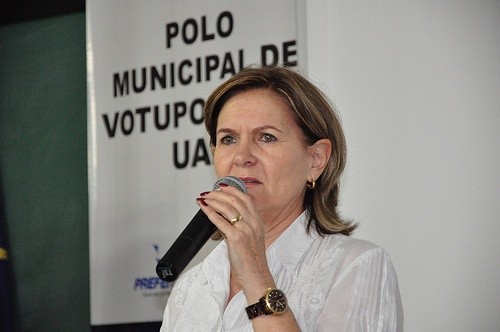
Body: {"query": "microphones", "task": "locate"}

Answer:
[155,175,248,283]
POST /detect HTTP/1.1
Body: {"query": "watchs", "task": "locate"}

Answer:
[245,286,289,320]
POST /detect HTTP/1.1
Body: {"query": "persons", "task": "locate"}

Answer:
[159,66,407,332]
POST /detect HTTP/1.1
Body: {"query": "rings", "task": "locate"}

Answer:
[230,213,242,225]
